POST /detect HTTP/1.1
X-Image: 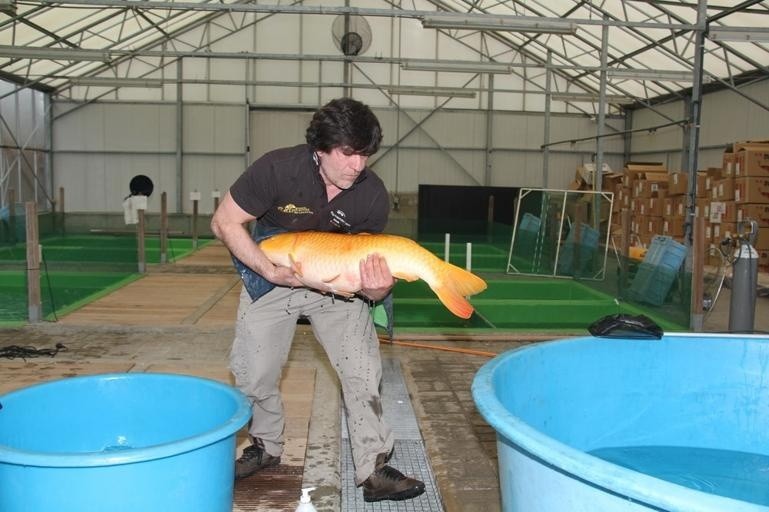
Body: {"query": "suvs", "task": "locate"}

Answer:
[0,373,252,512]
[471,332,769,512]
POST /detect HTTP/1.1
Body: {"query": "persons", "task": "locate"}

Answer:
[210,97,426,502]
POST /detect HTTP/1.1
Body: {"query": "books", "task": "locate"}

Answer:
[566,140,768,273]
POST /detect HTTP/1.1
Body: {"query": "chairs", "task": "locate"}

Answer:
[236,444,281,478]
[363,453,425,502]
